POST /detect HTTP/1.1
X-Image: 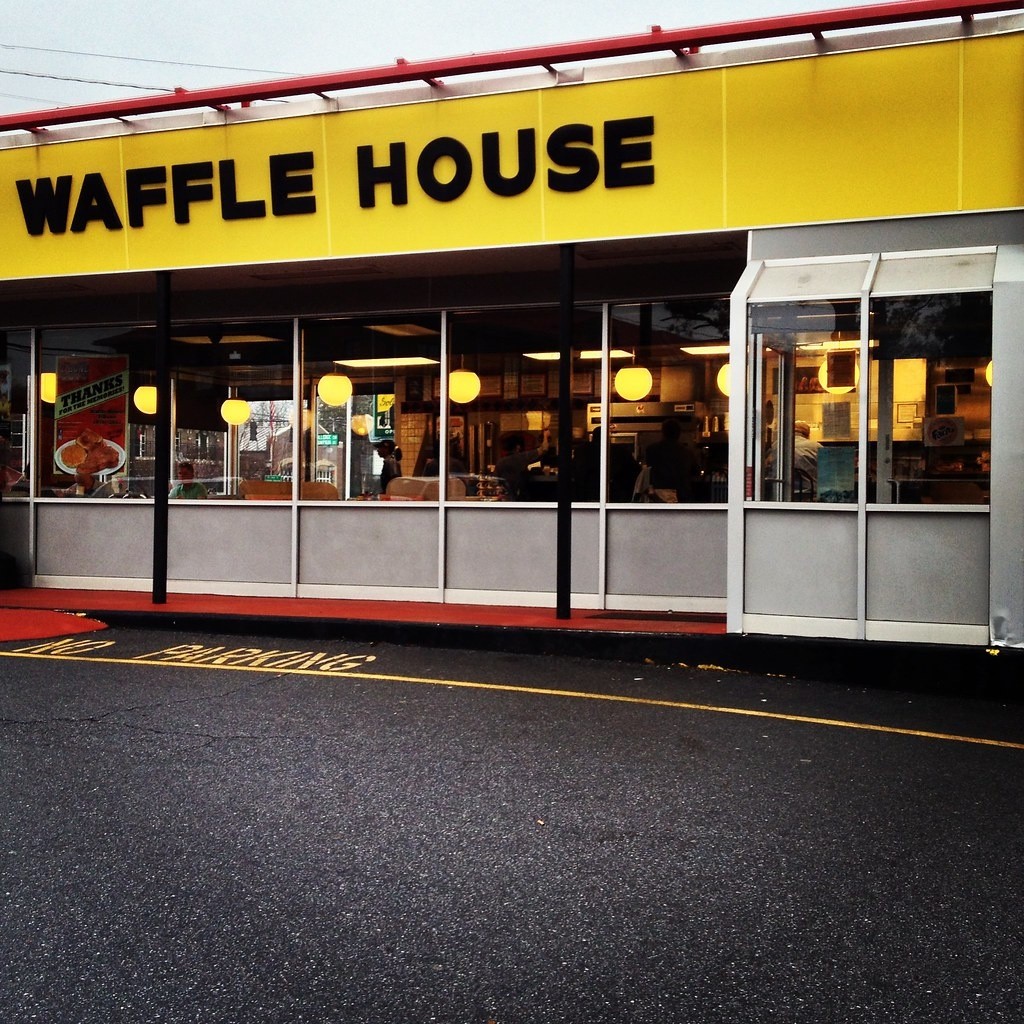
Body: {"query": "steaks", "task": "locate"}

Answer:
[77,443,117,474]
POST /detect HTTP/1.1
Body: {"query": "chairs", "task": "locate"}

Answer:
[790,468,814,502]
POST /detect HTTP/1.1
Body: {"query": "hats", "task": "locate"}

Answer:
[795,421,811,437]
[374,440,394,448]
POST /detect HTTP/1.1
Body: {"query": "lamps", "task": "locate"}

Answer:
[716,353,731,396]
[317,360,353,407]
[133,371,157,415]
[448,353,482,405]
[614,346,653,401]
[220,386,251,426]
[818,330,860,396]
[39,371,58,405]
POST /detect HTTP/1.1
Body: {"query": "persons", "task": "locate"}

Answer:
[55,462,116,499]
[167,463,208,499]
[418,418,729,502]
[374,440,403,495]
[763,420,826,500]
